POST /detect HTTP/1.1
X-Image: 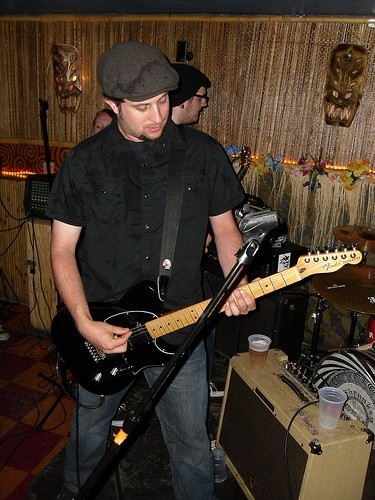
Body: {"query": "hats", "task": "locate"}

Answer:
[97,41,179,102]
[168,63,210,106]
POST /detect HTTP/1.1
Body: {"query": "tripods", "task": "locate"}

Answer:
[34,98,76,431]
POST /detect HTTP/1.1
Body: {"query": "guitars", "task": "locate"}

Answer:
[50,243,363,396]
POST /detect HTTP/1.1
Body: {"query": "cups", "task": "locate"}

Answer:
[212,448,227,483]
[248,334,272,369]
[318,387,347,430]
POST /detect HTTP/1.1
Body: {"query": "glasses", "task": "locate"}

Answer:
[195,94,210,103]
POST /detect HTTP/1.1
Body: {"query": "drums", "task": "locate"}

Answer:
[312,348,375,434]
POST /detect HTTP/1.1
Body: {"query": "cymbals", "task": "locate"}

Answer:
[311,263,375,315]
[332,225,375,254]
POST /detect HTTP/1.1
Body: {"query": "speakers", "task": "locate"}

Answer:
[203,259,309,373]
[216,348,373,500]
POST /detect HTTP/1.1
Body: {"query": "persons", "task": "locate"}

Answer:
[92,108,115,134]
[45,42,261,500]
[167,61,217,442]
